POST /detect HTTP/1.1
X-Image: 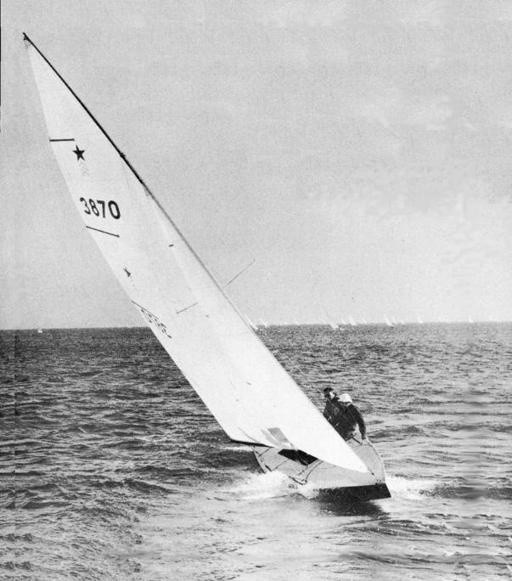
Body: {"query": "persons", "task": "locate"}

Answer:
[331,394,366,446]
[323,387,339,424]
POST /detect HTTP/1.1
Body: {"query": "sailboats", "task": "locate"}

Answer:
[22,31,395,501]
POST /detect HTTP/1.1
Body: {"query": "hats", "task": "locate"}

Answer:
[338,394,352,403]
